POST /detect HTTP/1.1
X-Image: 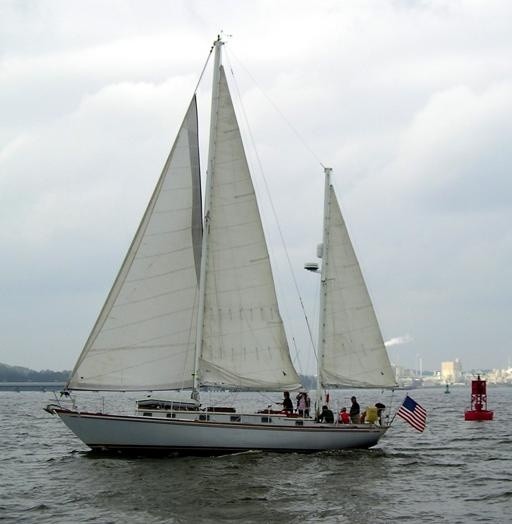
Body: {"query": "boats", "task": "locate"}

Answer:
[464,374,494,420]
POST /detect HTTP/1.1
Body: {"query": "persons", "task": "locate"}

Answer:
[275,390,311,417]
[317,396,385,426]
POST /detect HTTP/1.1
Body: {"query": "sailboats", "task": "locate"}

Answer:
[44,33,400,453]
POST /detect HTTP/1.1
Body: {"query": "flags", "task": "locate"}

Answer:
[397,396,426,433]
